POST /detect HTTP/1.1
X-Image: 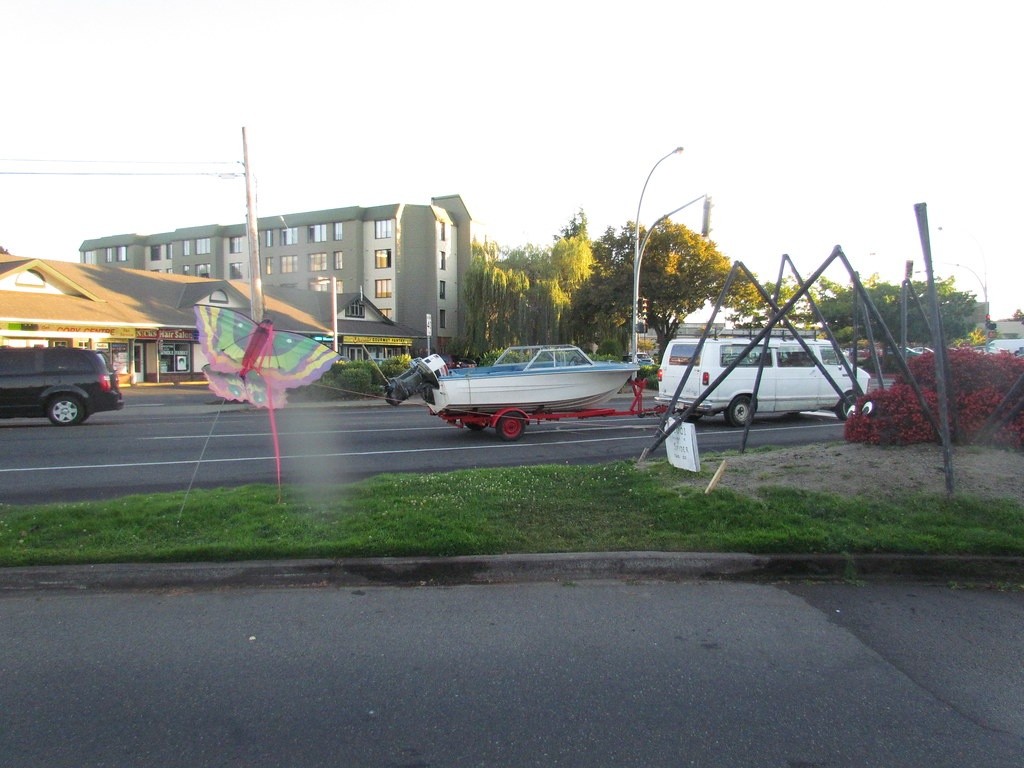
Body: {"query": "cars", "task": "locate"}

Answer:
[636,352,653,365]
[948,338,1024,360]
[436,353,476,368]
[841,337,934,374]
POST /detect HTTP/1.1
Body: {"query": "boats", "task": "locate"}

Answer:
[380,345,641,416]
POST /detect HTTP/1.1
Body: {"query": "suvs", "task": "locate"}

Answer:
[1,344,126,428]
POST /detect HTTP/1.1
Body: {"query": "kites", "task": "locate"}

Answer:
[194,304,342,504]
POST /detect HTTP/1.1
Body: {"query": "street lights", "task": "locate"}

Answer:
[629,144,687,392]
[954,263,991,353]
[216,171,265,326]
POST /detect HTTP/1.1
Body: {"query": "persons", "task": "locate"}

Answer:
[178,358,185,369]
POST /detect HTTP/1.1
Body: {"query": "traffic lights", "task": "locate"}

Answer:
[700,201,714,237]
[635,323,646,333]
[985,313,991,326]
[637,297,650,319]
[988,322,996,330]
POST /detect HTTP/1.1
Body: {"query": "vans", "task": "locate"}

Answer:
[650,327,872,429]
